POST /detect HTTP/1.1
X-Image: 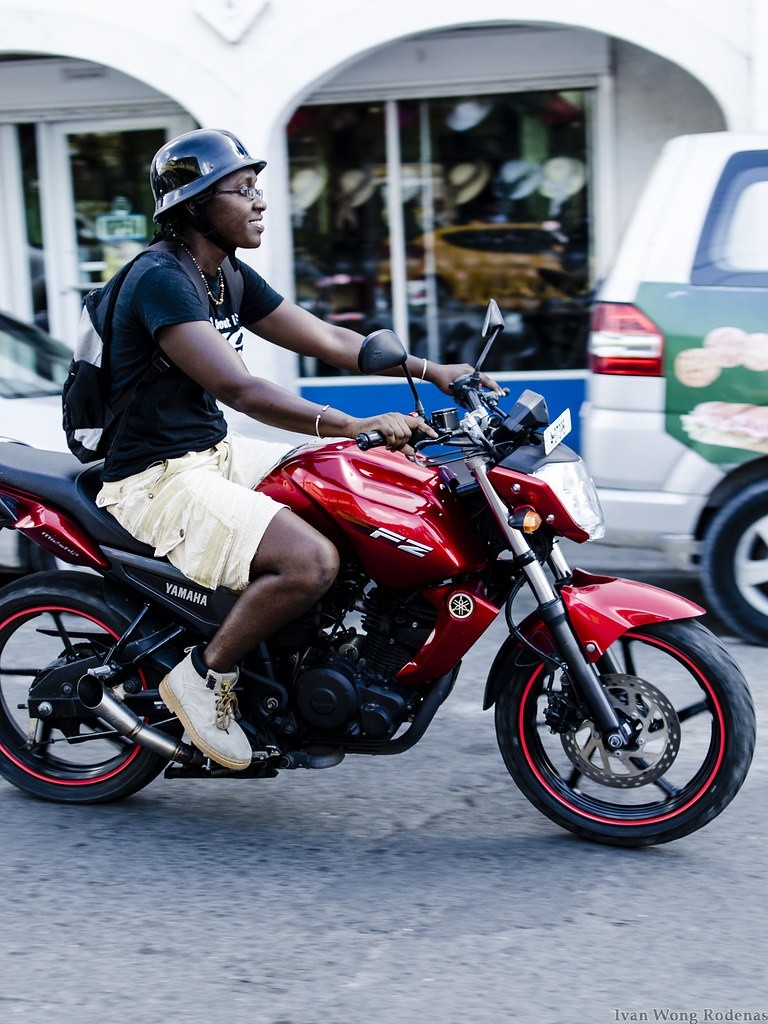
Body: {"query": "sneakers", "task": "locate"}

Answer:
[158,642,253,770]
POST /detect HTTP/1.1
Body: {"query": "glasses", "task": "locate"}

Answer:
[214,188,264,199]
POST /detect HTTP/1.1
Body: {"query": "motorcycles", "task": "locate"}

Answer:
[0,298,758,849]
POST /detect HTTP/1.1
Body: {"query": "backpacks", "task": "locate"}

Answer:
[61,242,244,464]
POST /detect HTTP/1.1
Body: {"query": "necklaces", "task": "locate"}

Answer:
[170,228,225,305]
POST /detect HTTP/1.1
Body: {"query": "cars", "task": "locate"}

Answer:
[381,220,567,312]
[0,313,78,456]
[579,127,768,648]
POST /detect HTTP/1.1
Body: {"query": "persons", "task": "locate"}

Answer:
[94,125,508,771]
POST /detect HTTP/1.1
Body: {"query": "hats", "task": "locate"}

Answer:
[150,129,268,226]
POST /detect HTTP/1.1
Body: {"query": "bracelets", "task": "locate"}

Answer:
[418,358,427,384]
[315,404,330,439]
